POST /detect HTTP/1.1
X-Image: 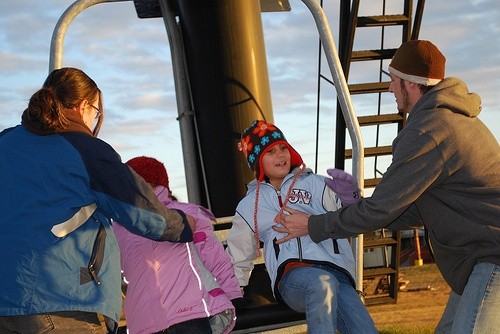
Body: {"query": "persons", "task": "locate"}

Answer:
[224,120,381,334]
[271,40,500,334]
[113,156,243,334]
[0,67,196,334]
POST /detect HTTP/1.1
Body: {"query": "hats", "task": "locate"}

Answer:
[127,156,169,189]
[388,40,446,86]
[241,120,306,258]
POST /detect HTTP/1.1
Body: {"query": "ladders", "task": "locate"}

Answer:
[335,0,425,305]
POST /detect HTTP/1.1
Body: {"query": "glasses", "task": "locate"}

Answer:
[87,102,102,119]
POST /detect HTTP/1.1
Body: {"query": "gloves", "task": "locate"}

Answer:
[324,169,361,208]
